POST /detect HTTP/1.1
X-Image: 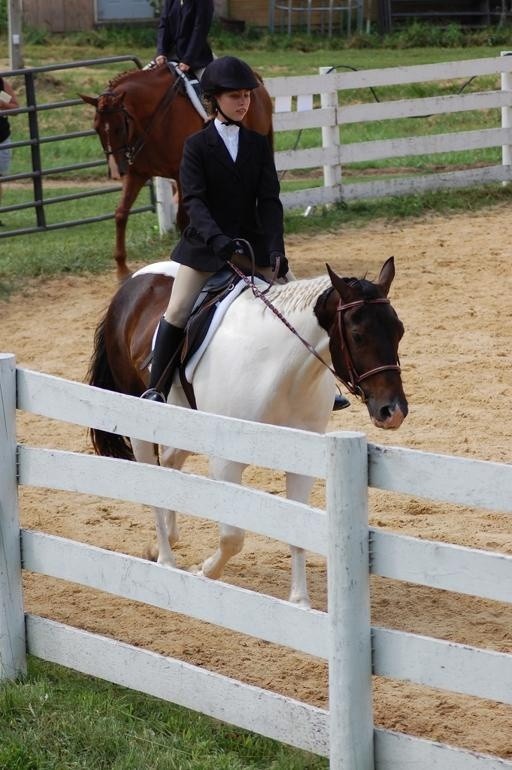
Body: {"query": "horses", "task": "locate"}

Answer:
[76,61,274,288]
[80,255,409,610]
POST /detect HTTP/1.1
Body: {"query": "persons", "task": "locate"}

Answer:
[0,77,20,226]
[154,0,217,83]
[144,56,351,411]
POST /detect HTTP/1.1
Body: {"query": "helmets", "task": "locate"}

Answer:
[199,56,260,93]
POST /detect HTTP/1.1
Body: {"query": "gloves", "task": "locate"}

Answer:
[272,254,289,278]
[210,235,237,259]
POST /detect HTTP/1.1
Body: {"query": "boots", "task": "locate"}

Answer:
[332,393,350,411]
[145,317,188,403]
